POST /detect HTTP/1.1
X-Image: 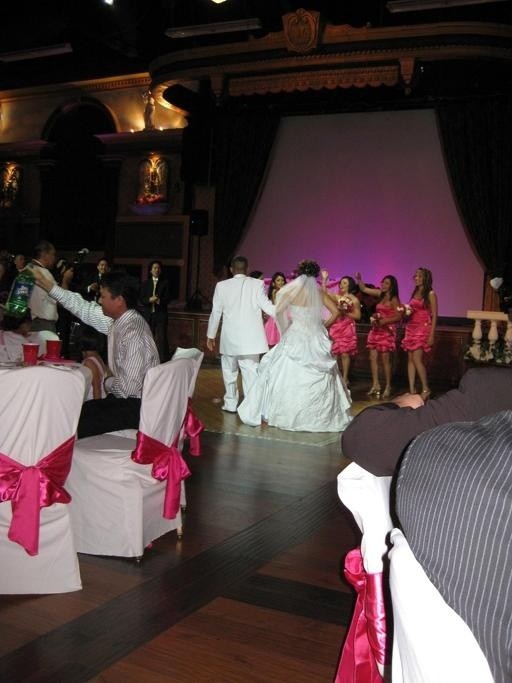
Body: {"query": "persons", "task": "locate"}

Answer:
[7,253,25,281]
[206,255,276,413]
[353,271,403,397]
[401,267,438,401]
[321,270,361,389]
[237,259,354,432]
[264,272,292,349]
[18,263,160,439]
[236,271,268,391]
[20,240,74,334]
[341,294,512,478]
[140,261,174,364]
[82,258,113,363]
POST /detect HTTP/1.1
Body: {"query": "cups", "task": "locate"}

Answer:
[46,340,62,362]
[21,343,40,368]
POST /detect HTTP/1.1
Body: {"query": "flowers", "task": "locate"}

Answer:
[370,312,394,336]
[397,303,413,340]
[337,296,353,320]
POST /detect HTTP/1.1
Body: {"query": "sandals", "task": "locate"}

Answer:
[382,387,392,398]
[367,388,381,395]
[421,391,431,400]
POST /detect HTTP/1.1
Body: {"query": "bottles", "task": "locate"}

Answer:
[4,259,35,317]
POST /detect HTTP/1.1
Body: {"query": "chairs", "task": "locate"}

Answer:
[1,330,29,361]
[387,527,494,683]
[336,461,393,683]
[105,346,205,511]
[1,365,85,594]
[63,357,193,562]
[30,330,60,357]
